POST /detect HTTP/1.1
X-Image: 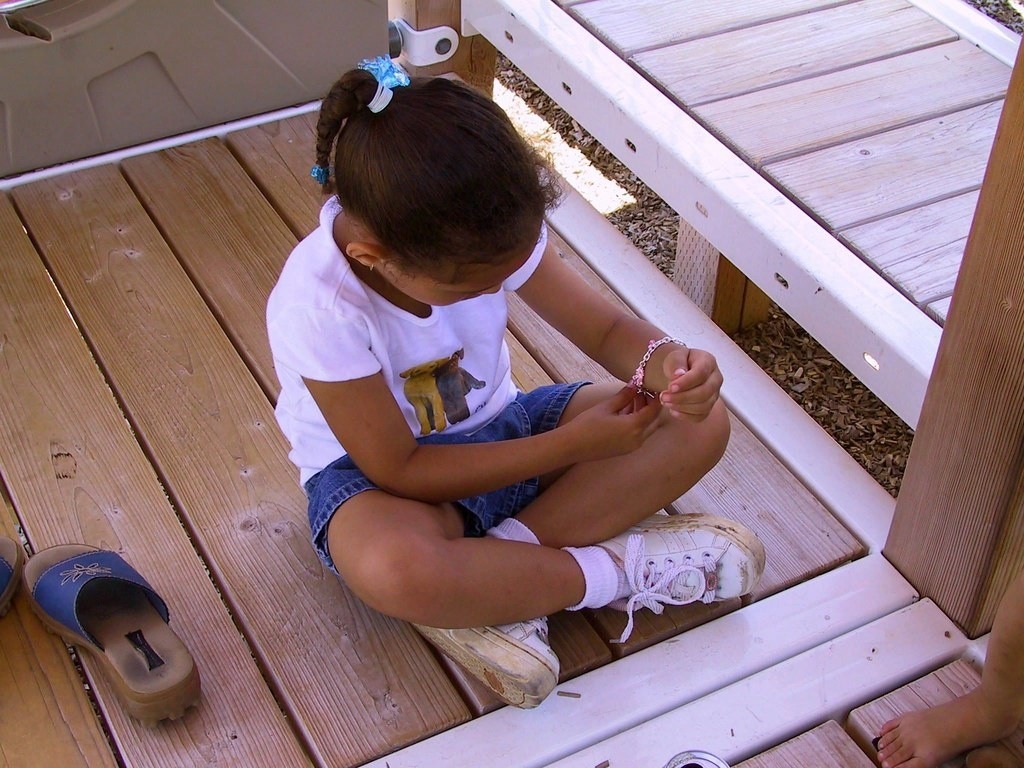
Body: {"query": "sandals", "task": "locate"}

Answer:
[0,536,26,617]
[16,541,201,729]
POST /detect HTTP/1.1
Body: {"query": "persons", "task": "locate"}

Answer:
[875,567,1024,767]
[264,66,767,712]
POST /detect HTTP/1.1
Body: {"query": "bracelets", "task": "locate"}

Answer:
[623,336,690,399]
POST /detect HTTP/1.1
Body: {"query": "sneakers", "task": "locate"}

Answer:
[595,513,767,615]
[410,614,559,710]
